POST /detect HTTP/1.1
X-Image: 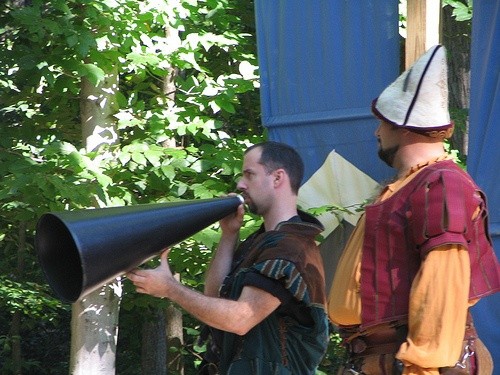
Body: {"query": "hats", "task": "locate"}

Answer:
[372,45,456,140]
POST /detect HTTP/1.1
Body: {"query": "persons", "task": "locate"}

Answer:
[327,44,500,375]
[125,142,326,375]
